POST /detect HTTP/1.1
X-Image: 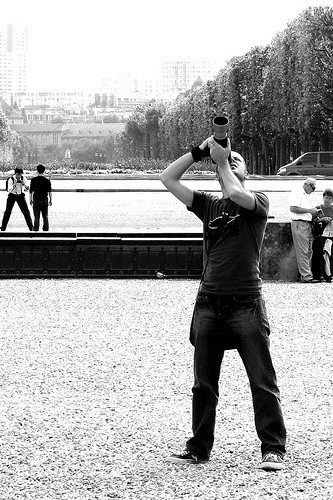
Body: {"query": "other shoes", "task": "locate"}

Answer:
[326,275,333,281]
[302,278,319,282]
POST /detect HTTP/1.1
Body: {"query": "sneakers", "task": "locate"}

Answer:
[261,452,284,469]
[166,449,208,464]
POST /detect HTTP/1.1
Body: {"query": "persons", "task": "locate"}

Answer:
[159,136,287,468]
[289,178,333,283]
[0,165,54,231]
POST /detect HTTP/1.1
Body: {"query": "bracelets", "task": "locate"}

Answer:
[191,145,202,162]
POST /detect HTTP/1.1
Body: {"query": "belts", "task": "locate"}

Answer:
[293,219,311,223]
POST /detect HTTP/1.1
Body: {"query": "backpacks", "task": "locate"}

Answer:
[6,176,23,192]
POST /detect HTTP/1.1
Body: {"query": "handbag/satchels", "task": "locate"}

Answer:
[189,301,237,350]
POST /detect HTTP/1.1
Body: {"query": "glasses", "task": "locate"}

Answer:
[310,184,315,190]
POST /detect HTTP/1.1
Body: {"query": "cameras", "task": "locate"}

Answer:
[212,116,231,164]
[17,177,22,183]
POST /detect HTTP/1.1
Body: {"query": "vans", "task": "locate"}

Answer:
[277,151,333,177]
